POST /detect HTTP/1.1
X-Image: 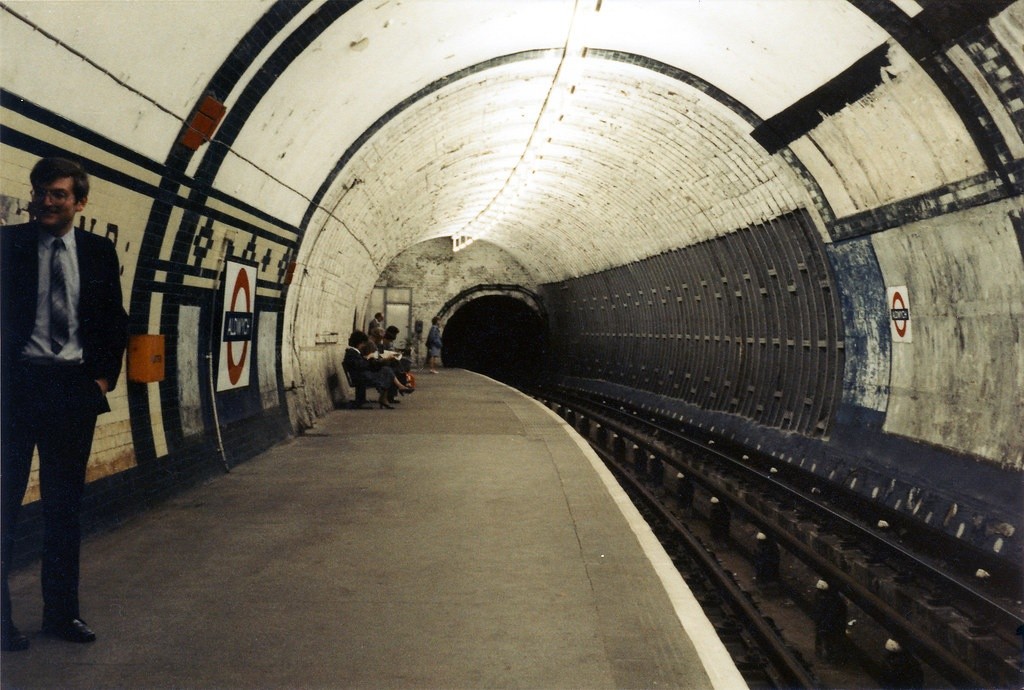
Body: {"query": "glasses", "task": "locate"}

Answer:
[30,187,67,208]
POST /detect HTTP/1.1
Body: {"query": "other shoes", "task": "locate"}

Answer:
[430,370,434,372]
[433,371,439,373]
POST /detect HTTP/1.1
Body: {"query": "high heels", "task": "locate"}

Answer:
[399,388,414,396]
[379,401,394,409]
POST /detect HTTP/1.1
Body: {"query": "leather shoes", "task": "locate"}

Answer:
[0,616,30,651]
[41,618,96,643]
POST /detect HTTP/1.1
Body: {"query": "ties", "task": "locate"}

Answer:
[50,238,70,355]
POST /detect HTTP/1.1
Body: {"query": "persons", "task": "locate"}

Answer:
[366,311,385,348]
[0,155,135,658]
[425,315,443,374]
[359,326,412,403]
[343,329,416,412]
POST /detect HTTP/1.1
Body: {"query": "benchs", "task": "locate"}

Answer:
[342,361,378,409]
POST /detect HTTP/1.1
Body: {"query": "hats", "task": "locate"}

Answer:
[385,326,399,340]
